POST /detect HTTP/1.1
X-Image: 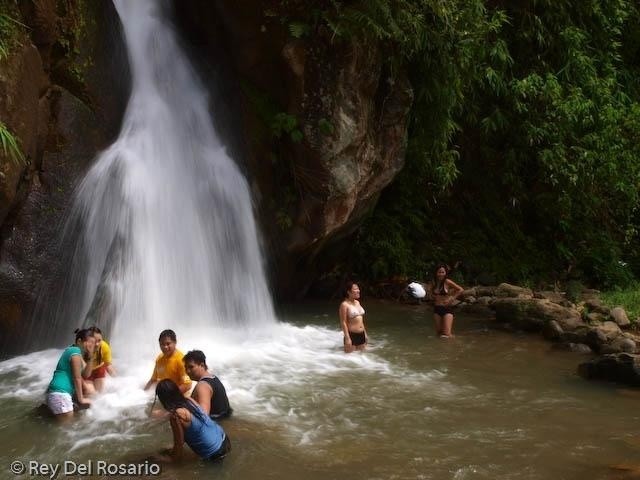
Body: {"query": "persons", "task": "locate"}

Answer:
[150,379,231,467]
[144,329,192,417]
[46,328,95,423]
[396,281,427,305]
[82,327,118,396]
[431,264,464,336]
[338,281,368,353]
[182,349,233,420]
